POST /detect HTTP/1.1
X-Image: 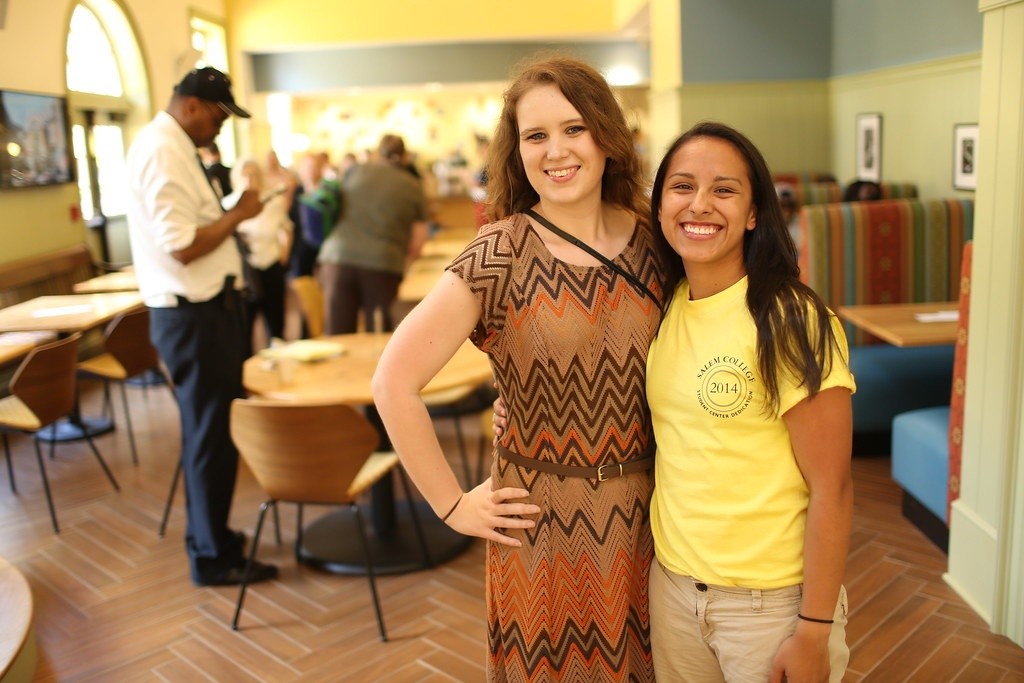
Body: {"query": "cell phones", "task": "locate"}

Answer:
[259,186,287,205]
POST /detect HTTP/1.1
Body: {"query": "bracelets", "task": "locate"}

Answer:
[442,494,463,522]
[798,614,835,624]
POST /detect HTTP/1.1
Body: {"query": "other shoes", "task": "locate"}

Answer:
[230,530,246,552]
[193,563,278,586]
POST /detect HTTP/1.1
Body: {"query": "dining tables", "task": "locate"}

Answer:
[235,219,494,579]
[0,267,160,443]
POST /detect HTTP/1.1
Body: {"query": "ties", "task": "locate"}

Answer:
[198,156,252,279]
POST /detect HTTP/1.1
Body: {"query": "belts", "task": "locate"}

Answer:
[497,441,655,482]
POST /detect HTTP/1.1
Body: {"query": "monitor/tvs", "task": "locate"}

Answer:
[0,90,76,190]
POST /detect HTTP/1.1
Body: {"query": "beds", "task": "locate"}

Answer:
[70,303,181,466]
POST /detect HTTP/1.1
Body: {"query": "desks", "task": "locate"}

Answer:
[835,302,958,346]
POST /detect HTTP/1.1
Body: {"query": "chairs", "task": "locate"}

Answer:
[155,353,185,537]
[228,398,435,640]
[0,330,121,535]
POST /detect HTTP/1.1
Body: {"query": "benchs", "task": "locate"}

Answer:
[759,174,973,556]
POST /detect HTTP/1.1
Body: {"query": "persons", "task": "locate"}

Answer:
[195,140,356,354]
[493,121,856,683]
[371,57,685,683]
[317,135,431,335]
[125,68,282,588]
[771,182,802,253]
[843,180,883,203]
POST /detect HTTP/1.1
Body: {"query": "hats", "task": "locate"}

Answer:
[175,66,252,119]
